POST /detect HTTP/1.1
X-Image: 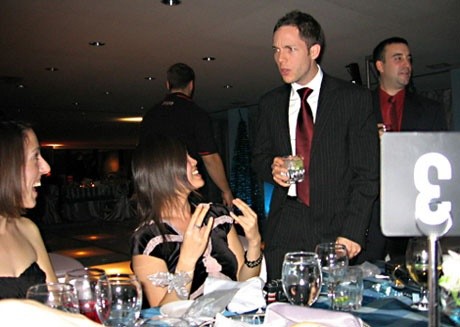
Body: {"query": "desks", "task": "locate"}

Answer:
[141,267,460,327]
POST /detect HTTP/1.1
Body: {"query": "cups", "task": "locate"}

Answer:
[315,243,349,295]
[328,267,364,312]
[281,251,323,307]
[60,267,112,325]
[93,273,143,327]
[281,157,305,183]
[26,282,80,315]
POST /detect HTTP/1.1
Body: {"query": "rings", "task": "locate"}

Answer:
[194,224,201,229]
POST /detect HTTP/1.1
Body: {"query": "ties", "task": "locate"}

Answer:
[388,97,399,132]
[296,87,314,207]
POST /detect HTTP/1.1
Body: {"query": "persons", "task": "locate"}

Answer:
[0,119,62,310]
[364,36,449,261]
[135,62,234,216]
[128,137,263,313]
[249,11,379,282]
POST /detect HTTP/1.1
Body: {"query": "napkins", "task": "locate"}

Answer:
[203,276,267,314]
[263,301,371,327]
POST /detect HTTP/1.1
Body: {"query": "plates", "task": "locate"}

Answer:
[159,299,195,318]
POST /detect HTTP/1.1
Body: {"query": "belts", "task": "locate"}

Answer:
[287,195,304,204]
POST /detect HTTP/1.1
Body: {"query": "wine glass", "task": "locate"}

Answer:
[405,238,443,311]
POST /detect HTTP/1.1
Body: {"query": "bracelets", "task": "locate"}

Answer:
[244,249,263,268]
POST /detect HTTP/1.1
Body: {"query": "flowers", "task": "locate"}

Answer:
[438,250,460,307]
